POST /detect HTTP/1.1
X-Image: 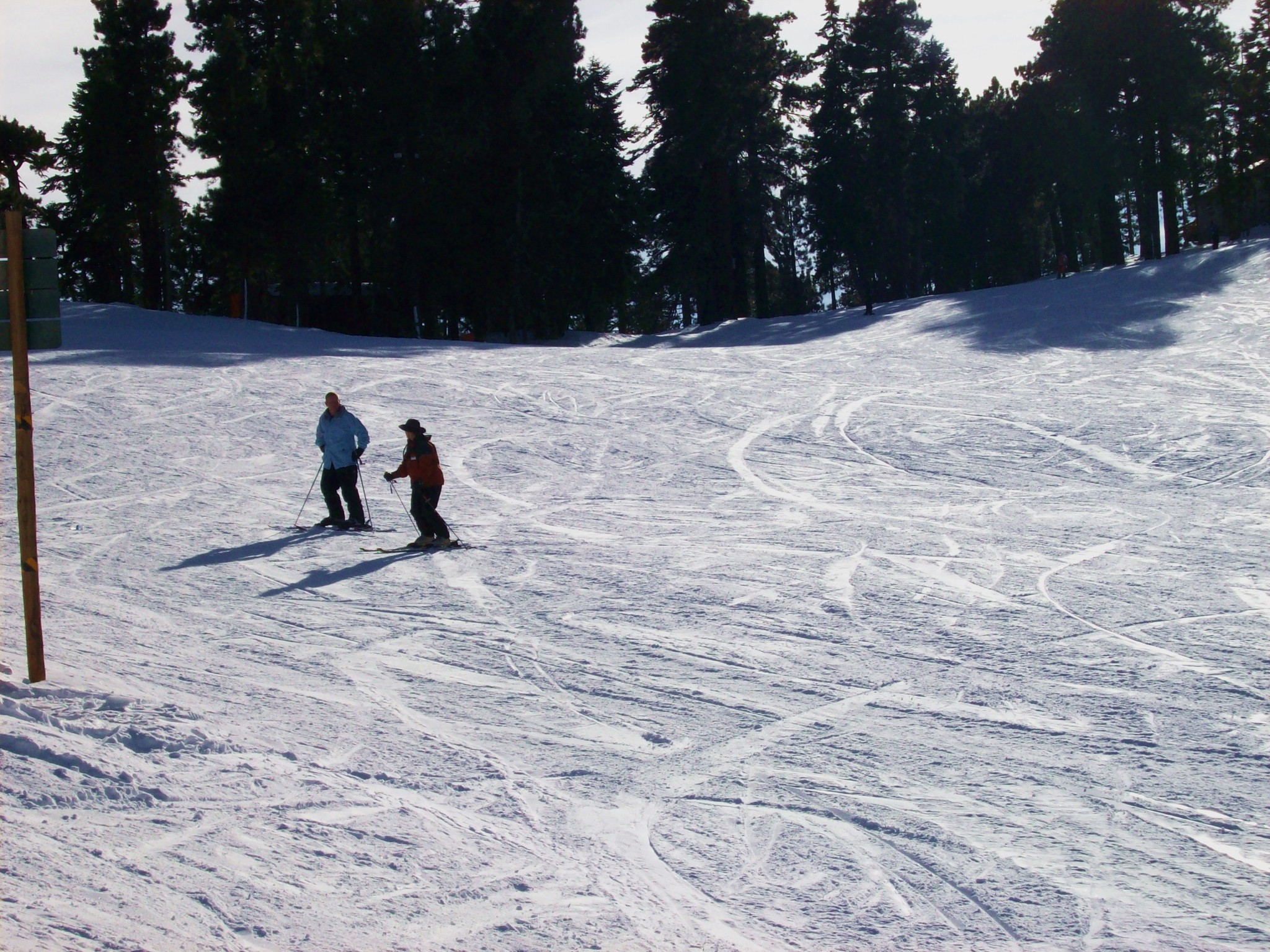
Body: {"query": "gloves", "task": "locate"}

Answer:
[411,482,422,490]
[351,448,364,460]
[383,472,392,482]
[320,445,324,453]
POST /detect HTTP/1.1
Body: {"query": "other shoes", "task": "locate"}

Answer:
[339,520,363,529]
[433,536,449,549]
[321,516,345,525]
[415,535,434,547]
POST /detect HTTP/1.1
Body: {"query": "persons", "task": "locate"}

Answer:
[385,419,450,550]
[315,391,370,529]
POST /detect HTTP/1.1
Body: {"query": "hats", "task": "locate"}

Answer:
[398,419,426,433]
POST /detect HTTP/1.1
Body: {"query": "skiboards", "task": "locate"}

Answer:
[359,542,487,553]
[267,524,398,533]
